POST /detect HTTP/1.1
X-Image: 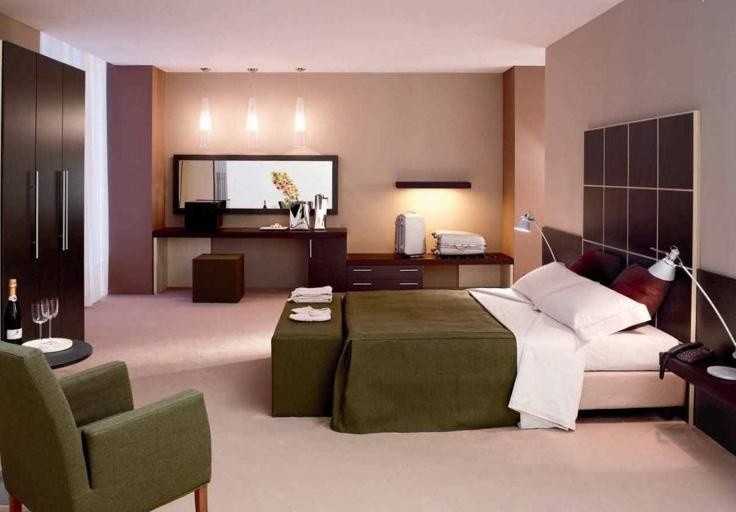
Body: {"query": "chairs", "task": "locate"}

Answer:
[0,340,212,512]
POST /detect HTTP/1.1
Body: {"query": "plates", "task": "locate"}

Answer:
[259,226,288,230]
[22,338,74,353]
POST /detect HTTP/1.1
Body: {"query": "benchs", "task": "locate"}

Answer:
[272,294,343,417]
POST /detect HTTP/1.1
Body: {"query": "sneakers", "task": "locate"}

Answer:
[290,305,332,322]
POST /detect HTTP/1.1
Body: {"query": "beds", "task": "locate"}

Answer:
[332,288,688,435]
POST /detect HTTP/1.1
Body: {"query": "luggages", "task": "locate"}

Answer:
[431,232,487,256]
[393,210,425,257]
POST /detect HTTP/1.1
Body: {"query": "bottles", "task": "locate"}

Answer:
[263,200,267,208]
[2,279,23,345]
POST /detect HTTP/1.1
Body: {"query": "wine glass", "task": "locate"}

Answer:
[31,303,49,345]
[40,298,59,345]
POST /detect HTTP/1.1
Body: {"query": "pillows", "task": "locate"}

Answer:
[512,251,670,340]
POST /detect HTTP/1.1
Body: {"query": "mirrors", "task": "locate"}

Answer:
[173,155,338,215]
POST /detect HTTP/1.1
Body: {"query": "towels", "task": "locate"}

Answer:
[287,286,333,304]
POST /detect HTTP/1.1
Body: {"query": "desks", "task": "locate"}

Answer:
[152,227,347,292]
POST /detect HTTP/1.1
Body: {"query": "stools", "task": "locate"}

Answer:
[193,254,245,302]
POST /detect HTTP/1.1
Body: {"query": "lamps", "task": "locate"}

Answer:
[648,243,736,347]
[514,210,556,261]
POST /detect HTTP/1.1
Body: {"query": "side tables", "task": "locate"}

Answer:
[20,338,93,369]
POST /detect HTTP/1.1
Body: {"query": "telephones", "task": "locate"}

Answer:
[668,341,715,364]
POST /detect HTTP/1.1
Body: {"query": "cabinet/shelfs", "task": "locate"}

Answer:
[4,39,85,344]
[347,253,514,288]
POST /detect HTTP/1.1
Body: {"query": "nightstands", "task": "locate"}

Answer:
[658,350,736,456]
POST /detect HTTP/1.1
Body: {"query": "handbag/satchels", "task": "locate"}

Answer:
[289,199,311,230]
[314,194,328,229]
